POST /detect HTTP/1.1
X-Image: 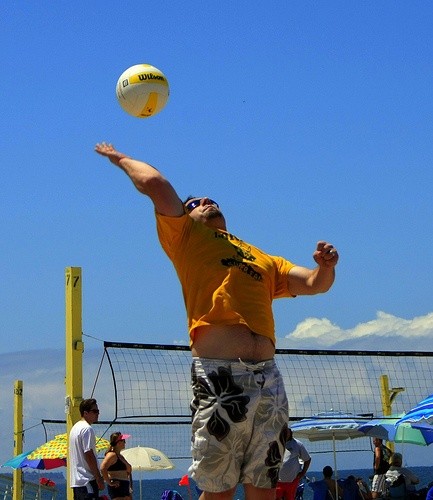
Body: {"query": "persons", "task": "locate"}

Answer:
[386,452,420,500]
[68,398,104,500]
[372,437,392,500]
[99,431,135,500]
[276,428,311,500]
[92,139,338,500]
[322,465,371,500]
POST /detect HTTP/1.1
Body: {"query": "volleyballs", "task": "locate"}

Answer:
[114,63,170,119]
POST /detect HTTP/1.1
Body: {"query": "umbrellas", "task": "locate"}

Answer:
[395,393,433,446]
[357,409,407,441]
[0,432,111,471]
[119,445,175,500]
[289,408,370,500]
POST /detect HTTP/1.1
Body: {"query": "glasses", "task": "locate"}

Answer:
[186,199,219,213]
[89,409,99,414]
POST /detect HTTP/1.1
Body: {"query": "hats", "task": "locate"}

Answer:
[114,434,130,443]
